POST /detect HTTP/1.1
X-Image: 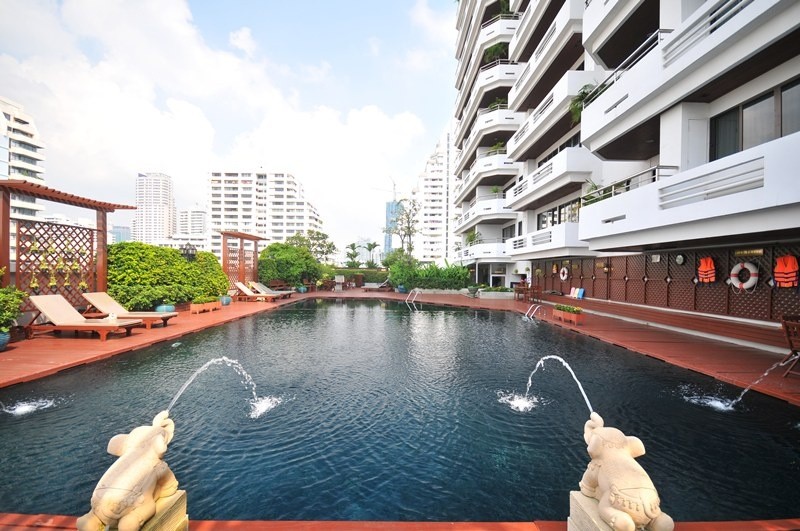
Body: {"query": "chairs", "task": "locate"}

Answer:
[360,278,391,292]
[514,284,543,305]
[779,315,800,377]
[270,278,316,292]
[82,292,179,329]
[230,281,296,303]
[23,294,142,341]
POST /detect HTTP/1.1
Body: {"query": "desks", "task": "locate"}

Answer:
[83,313,109,319]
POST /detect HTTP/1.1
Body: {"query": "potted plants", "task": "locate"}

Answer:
[468,282,479,294]
[190,295,222,315]
[0,285,30,351]
[552,304,587,327]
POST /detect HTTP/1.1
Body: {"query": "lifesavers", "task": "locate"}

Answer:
[730,263,758,290]
[560,267,568,280]
